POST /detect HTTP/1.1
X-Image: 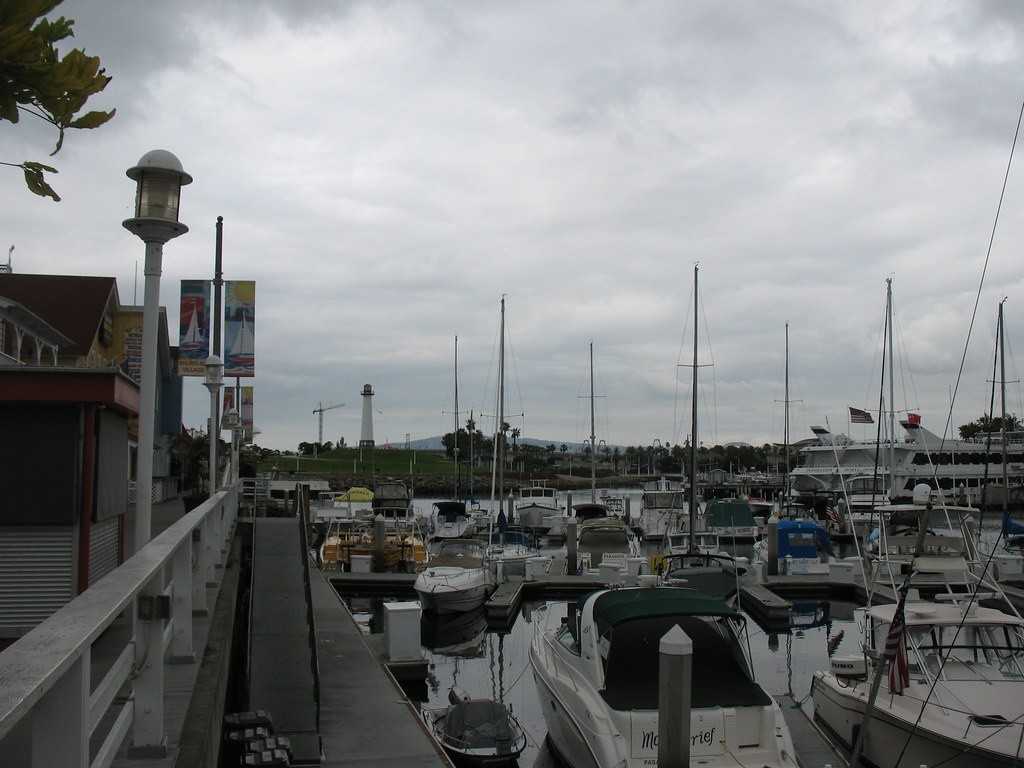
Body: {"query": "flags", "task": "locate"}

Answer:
[908,413,921,424]
[825,504,842,525]
[849,407,874,423]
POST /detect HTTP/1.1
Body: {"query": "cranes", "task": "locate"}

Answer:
[313,401,346,445]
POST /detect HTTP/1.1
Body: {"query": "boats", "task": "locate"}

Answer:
[525,516,801,768]
[423,685,529,764]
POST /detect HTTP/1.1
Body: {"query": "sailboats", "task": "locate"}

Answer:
[316,260,1024,768]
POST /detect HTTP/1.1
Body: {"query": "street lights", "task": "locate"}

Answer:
[117,147,193,554]
[227,407,240,483]
[200,355,227,499]
